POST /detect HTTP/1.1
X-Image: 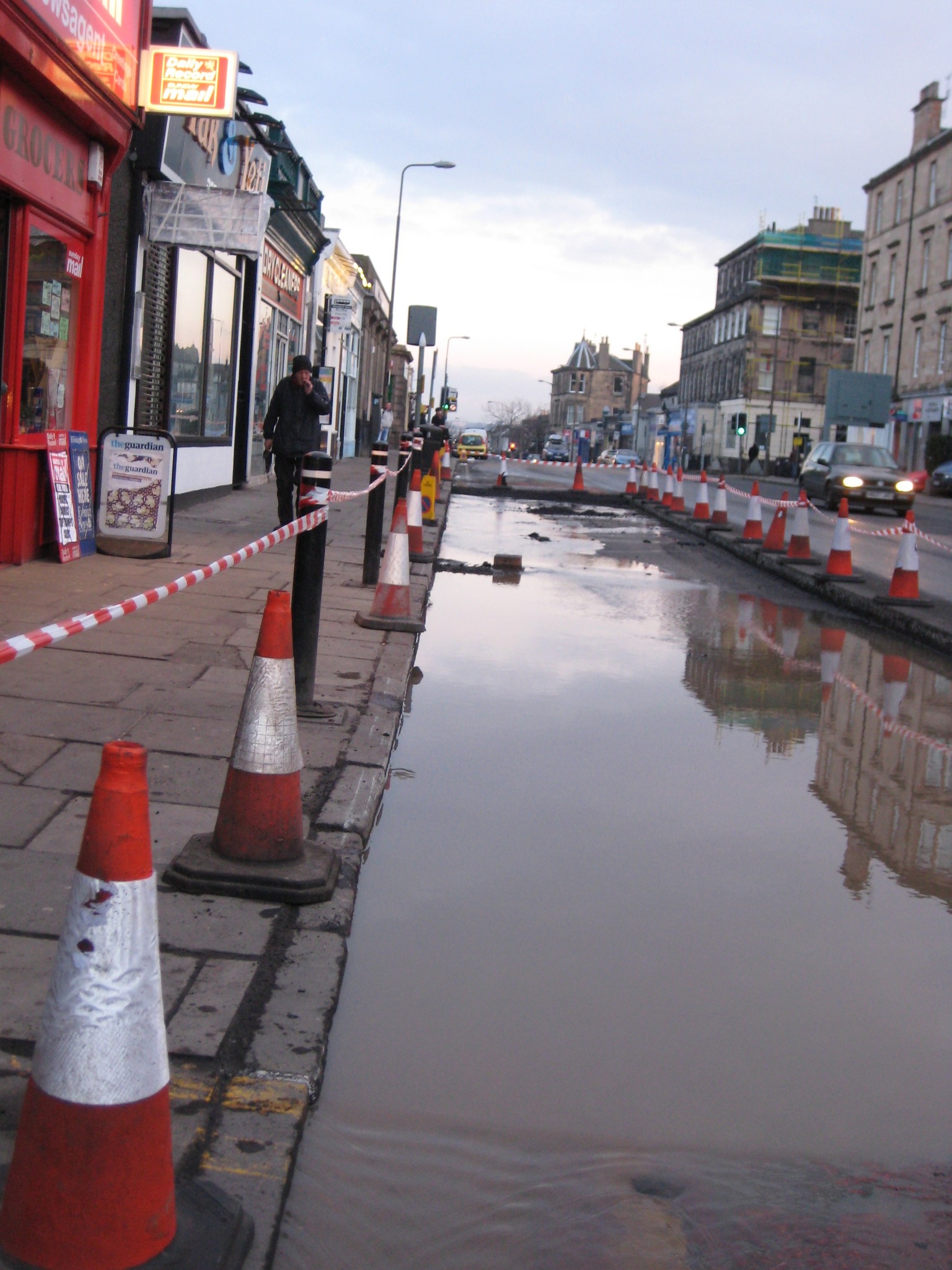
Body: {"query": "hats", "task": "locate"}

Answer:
[436,407,442,411]
[291,355,312,373]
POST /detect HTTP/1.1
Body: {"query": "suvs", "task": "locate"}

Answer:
[542,435,570,462]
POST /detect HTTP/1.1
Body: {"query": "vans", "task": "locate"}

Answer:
[457,429,488,460]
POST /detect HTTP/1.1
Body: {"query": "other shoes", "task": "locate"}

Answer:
[273,523,287,532]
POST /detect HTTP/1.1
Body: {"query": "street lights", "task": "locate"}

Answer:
[622,346,641,455]
[382,161,461,414]
[443,335,470,424]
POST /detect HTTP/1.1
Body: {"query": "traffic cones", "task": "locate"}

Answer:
[625,460,685,516]
[420,443,467,528]
[753,489,791,555]
[780,487,823,564]
[379,469,436,564]
[568,456,589,493]
[703,473,732,531]
[813,496,866,584]
[1,737,255,1270]
[873,509,932,605]
[733,480,767,544]
[686,469,713,523]
[354,497,429,633]
[161,589,342,911]
[736,594,914,740]
[497,450,508,487]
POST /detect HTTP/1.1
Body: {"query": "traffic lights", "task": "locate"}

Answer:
[731,413,749,436]
[509,443,515,451]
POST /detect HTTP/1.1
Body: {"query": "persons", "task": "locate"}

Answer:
[376,401,394,441]
[432,407,446,428]
[788,446,801,479]
[749,442,759,466]
[262,355,331,535]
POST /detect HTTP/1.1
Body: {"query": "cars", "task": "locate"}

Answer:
[800,441,916,515]
[929,461,952,498]
[595,449,642,465]
